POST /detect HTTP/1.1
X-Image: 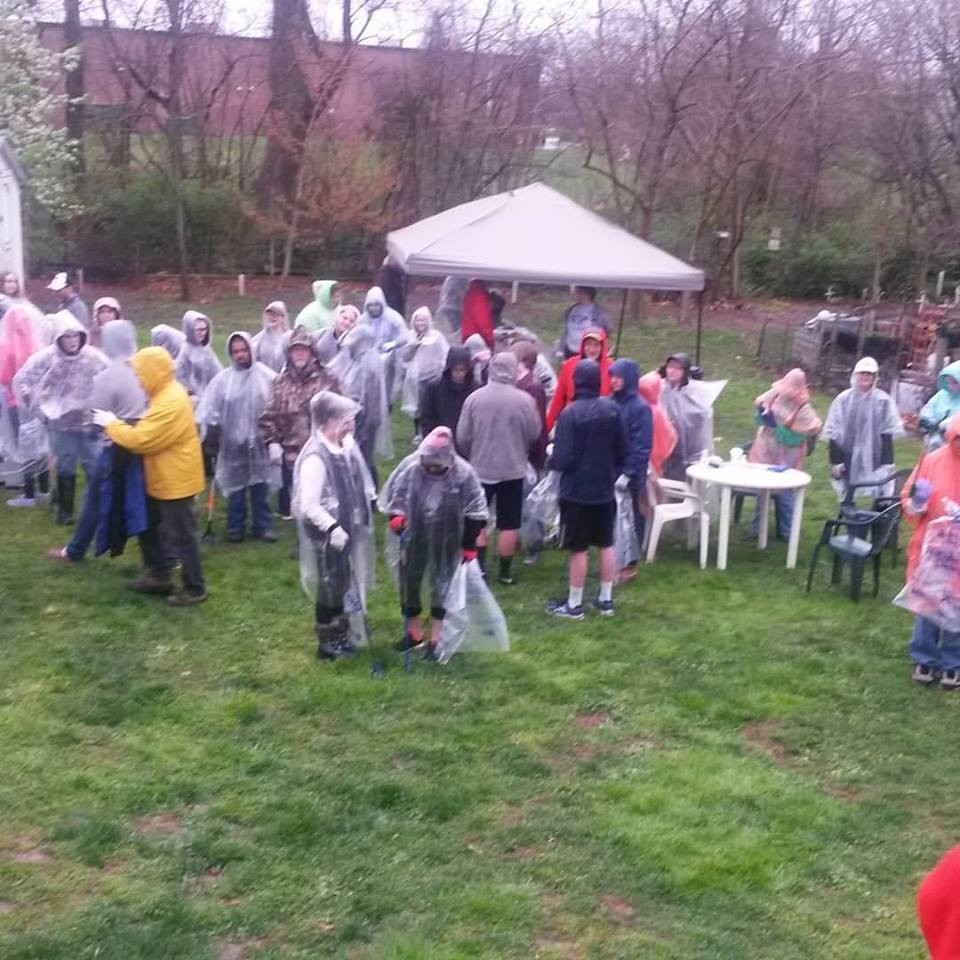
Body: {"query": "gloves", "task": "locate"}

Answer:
[463,548,479,562]
[268,442,284,466]
[90,408,119,429]
[829,462,847,481]
[912,478,933,505]
[330,525,350,554]
[390,515,409,534]
[614,473,632,491]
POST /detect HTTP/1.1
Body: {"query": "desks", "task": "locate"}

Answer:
[686,462,811,570]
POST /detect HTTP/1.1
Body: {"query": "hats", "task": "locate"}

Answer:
[582,326,607,342]
[855,357,878,373]
[46,272,74,291]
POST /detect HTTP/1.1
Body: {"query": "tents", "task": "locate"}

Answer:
[386,182,705,366]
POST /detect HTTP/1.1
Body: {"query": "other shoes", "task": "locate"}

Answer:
[5,491,209,605]
[391,632,426,655]
[939,667,960,687]
[911,663,936,682]
[421,640,440,665]
[224,509,295,544]
[480,546,640,620]
[736,524,903,553]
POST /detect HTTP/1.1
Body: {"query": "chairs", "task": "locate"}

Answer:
[833,469,914,568]
[640,466,710,570]
[732,440,780,540]
[806,496,903,603]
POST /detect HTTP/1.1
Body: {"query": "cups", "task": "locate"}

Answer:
[730,447,744,468]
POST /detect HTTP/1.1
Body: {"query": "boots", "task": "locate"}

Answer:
[314,616,346,661]
[337,616,358,655]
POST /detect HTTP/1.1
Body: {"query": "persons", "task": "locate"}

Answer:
[0,272,960,606]
[902,412,960,687]
[546,357,627,622]
[378,426,488,662]
[291,392,377,659]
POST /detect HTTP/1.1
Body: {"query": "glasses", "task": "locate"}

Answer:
[196,327,208,331]
[98,309,113,313]
